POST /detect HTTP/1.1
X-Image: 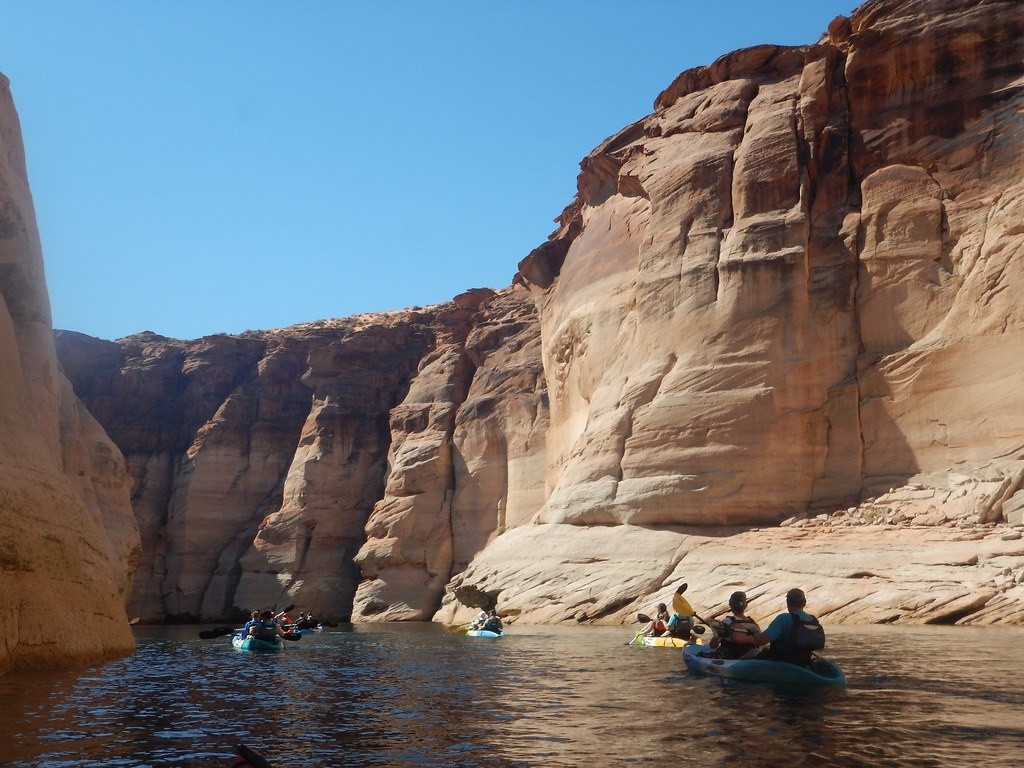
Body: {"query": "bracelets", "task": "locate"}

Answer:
[753,631,758,634]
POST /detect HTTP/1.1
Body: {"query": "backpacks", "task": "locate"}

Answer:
[488,618,499,632]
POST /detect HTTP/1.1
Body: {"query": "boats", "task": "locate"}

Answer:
[466,628,503,638]
[294,629,314,634]
[682,643,847,687]
[232,633,285,650]
[636,628,705,648]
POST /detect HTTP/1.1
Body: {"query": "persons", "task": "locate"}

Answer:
[639,604,670,638]
[747,588,825,671]
[472,611,487,631]
[295,611,308,630]
[244,609,297,643]
[305,612,318,629]
[480,610,503,635]
[660,611,694,640]
[705,592,761,660]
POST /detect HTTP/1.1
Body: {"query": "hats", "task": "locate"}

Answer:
[655,603,666,611]
[478,611,486,618]
[785,588,805,605]
[729,592,746,605]
[491,609,496,615]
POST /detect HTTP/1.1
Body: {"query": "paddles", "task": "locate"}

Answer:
[197,603,342,643]
[710,618,760,634]
[447,607,522,636]
[671,591,711,630]
[623,582,688,648]
[636,613,706,635]
